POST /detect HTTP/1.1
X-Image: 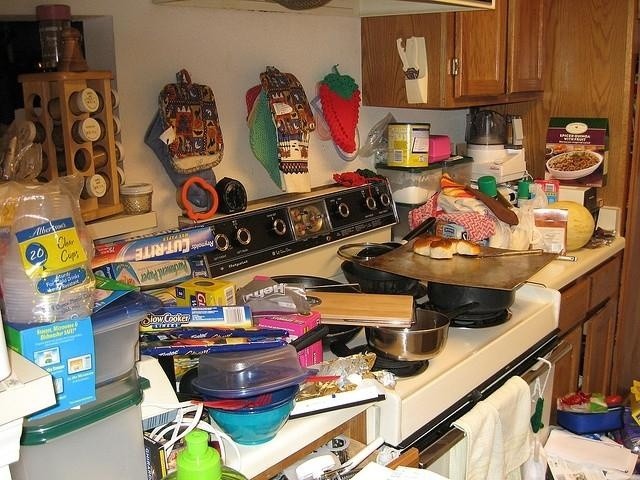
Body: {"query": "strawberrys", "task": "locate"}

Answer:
[320,66,360,153]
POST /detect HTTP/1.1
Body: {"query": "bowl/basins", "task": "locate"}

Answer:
[546,149,603,183]
[199,383,299,446]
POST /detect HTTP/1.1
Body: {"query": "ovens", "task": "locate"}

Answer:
[378,341,558,480]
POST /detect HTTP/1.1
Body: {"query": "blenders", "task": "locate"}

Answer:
[461,108,526,182]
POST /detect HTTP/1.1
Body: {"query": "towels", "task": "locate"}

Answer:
[408,172,534,252]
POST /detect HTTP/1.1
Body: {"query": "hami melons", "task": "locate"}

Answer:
[547,202,596,251]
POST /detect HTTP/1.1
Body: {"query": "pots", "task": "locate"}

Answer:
[269,218,529,365]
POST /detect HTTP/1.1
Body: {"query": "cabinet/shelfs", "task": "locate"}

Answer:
[547,244,624,429]
[245,411,420,480]
[360,0,551,110]
[475,1,640,401]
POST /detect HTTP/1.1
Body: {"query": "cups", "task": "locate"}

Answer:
[2,191,96,324]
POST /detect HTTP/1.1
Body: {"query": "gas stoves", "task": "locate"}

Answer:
[211,227,563,444]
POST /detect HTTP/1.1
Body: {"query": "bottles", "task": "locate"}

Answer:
[478,176,499,201]
[175,431,225,480]
[517,181,534,200]
[34,4,88,71]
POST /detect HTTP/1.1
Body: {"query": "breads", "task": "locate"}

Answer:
[413,236,480,259]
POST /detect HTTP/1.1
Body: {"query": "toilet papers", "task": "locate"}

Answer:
[0,310,11,383]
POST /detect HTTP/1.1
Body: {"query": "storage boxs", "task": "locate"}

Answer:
[8,288,165,479]
